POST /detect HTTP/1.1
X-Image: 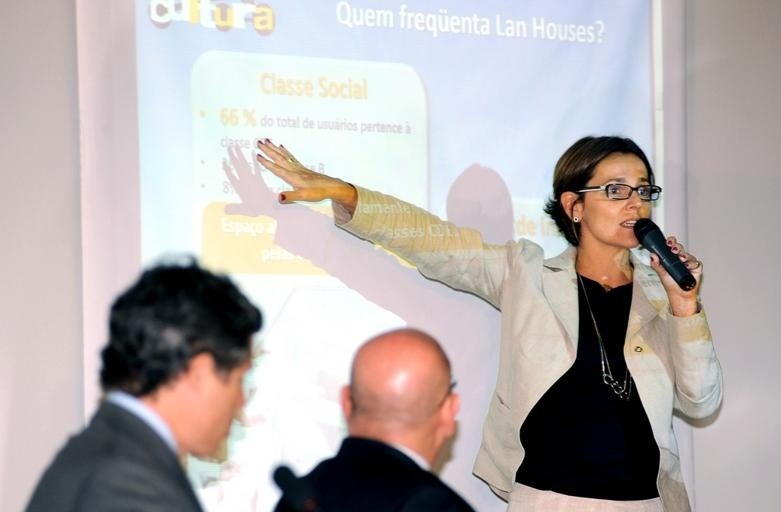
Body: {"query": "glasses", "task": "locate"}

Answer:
[576,183,662,201]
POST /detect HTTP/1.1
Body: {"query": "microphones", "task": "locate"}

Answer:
[633,217,697,292]
[270,465,320,512]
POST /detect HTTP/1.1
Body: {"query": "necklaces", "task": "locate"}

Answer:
[576,271,632,400]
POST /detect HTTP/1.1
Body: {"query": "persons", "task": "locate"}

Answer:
[254,134,724,512]
[272,329,477,512]
[25,266,262,512]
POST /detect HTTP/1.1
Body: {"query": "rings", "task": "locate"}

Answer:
[288,158,294,164]
[695,261,700,269]
